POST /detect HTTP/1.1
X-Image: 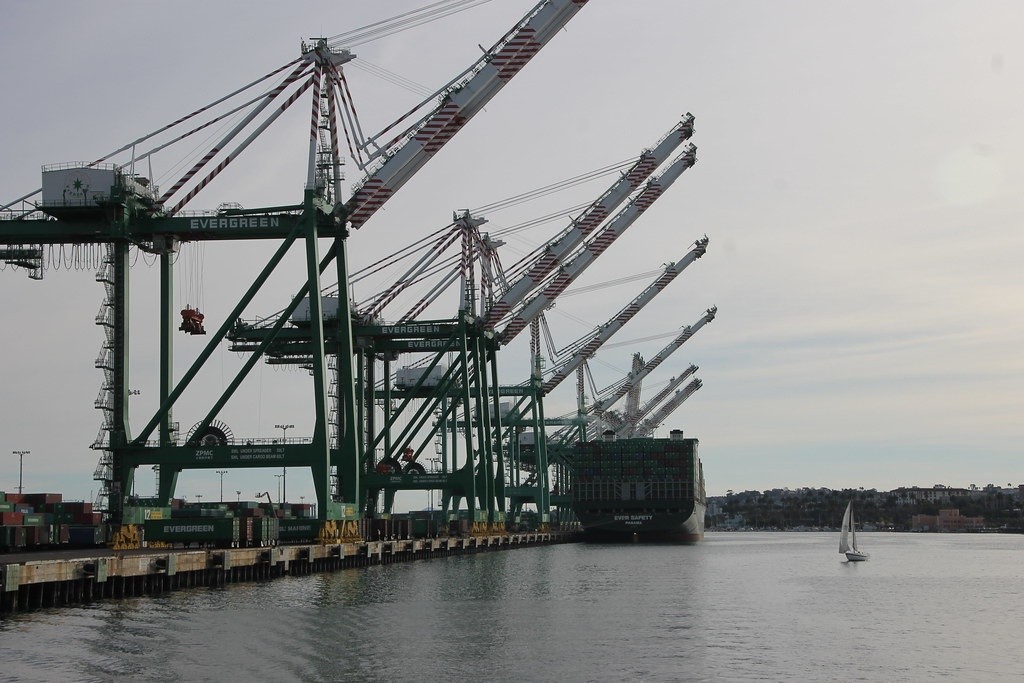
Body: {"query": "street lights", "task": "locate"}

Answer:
[195,495,203,503]
[12,450,30,494]
[215,470,228,502]
[273,474,284,504]
[275,424,295,501]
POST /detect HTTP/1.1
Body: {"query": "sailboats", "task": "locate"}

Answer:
[839,496,871,561]
